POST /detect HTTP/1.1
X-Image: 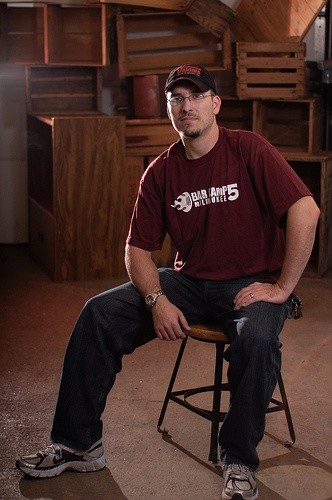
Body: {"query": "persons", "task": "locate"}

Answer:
[15,64,320,500]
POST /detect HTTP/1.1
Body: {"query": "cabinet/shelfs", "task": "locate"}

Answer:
[0,0,332,282]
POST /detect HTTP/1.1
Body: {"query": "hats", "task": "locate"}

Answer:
[165,64,216,95]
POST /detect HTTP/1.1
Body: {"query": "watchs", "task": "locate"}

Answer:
[145,290,165,307]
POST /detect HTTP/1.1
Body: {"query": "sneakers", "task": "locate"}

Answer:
[222,464,259,500]
[15,438,106,478]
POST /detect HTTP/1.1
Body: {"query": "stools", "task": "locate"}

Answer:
[156,324,296,467]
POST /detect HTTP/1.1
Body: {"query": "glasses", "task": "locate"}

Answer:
[166,94,213,106]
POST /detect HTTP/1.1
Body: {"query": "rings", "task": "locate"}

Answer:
[250,293,254,298]
